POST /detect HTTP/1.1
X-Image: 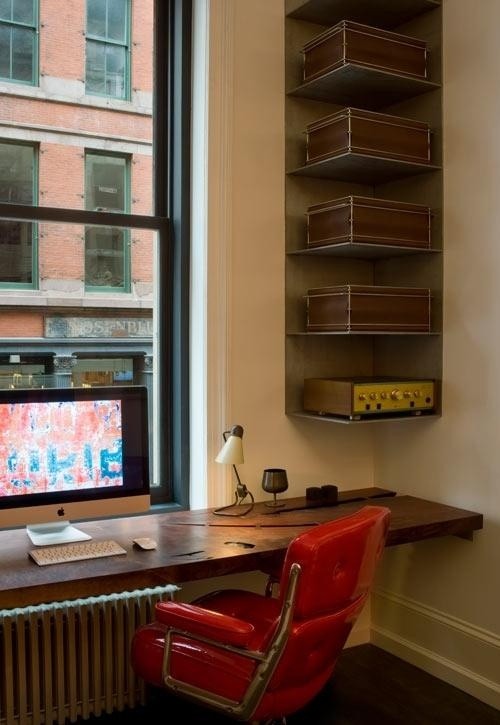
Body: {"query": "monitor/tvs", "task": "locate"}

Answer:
[0,385,151,546]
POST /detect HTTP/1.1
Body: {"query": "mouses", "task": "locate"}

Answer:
[133,537,157,551]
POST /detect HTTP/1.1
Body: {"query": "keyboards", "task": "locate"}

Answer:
[27,540,127,567]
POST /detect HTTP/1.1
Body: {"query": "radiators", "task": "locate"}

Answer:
[0,583,181,725]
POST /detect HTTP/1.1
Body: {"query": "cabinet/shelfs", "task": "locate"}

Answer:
[284,0,444,423]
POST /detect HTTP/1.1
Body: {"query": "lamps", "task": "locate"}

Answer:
[215,428,253,518]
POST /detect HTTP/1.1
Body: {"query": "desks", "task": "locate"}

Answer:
[0,488,483,616]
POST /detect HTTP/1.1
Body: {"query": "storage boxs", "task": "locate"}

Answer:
[300,19,430,81]
[304,106,432,164]
[303,285,432,331]
[305,194,432,248]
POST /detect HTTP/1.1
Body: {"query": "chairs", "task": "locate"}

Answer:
[131,504,393,725]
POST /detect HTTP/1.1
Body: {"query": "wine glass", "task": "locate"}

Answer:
[263,467,288,507]
